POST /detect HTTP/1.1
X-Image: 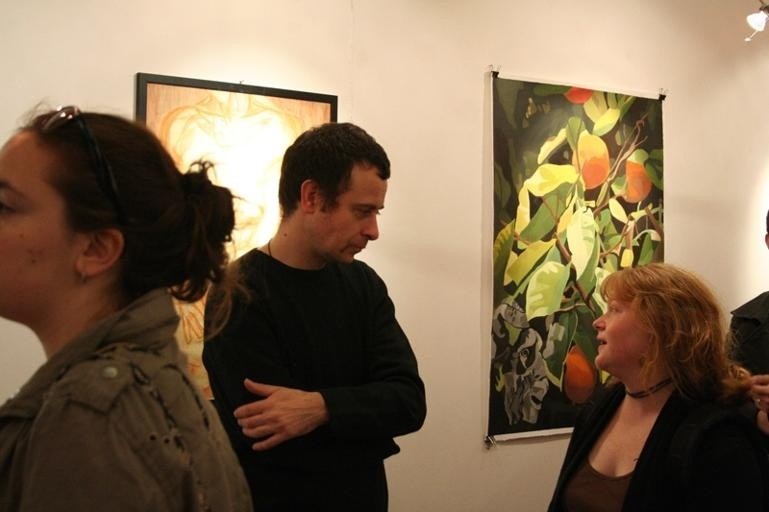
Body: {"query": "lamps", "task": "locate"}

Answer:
[747,5,769,31]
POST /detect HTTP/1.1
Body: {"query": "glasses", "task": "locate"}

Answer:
[42,105,126,221]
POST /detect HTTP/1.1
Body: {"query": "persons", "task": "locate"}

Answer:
[535,263,768,512]
[201,117,429,511]
[727,207,769,432]
[1,101,261,509]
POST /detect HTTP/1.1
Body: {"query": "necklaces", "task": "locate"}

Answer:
[624,373,681,398]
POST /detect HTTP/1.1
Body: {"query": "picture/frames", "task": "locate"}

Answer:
[136,72,338,409]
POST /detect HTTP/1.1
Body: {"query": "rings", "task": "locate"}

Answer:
[755,398,760,405]
